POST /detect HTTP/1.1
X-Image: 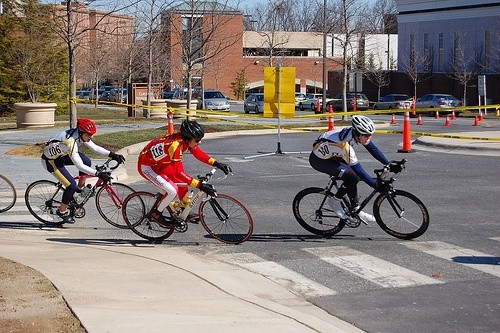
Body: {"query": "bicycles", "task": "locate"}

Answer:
[25,158,146,229]
[121,166,254,245]
[0,174,17,213]
[292,159,430,239]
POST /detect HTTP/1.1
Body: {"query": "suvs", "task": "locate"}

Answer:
[326,93,369,112]
[298,93,333,111]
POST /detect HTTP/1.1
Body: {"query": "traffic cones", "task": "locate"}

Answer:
[472,115,480,126]
[450,109,456,120]
[416,114,423,125]
[478,111,484,121]
[443,115,451,126]
[390,114,397,124]
[434,111,440,119]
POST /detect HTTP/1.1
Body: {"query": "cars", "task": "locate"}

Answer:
[295,92,309,107]
[162,89,196,99]
[196,90,230,112]
[415,94,463,117]
[244,93,264,114]
[76,86,127,103]
[372,94,413,110]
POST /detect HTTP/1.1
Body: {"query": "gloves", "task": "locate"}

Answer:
[375,181,390,195]
[95,170,113,182]
[198,182,217,197]
[108,151,125,164]
[212,160,232,176]
[389,162,402,174]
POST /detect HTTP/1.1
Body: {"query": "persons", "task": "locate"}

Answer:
[137,118,233,228]
[309,114,406,222]
[40,118,125,223]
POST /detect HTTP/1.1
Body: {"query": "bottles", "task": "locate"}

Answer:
[75,184,92,204]
[179,190,193,208]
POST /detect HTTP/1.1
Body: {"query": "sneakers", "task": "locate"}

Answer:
[147,208,170,228]
[176,208,205,220]
[77,186,96,196]
[327,198,346,219]
[351,207,376,222]
[56,209,75,224]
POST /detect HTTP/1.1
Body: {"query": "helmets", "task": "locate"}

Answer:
[77,118,96,134]
[180,118,205,140]
[351,114,375,135]
